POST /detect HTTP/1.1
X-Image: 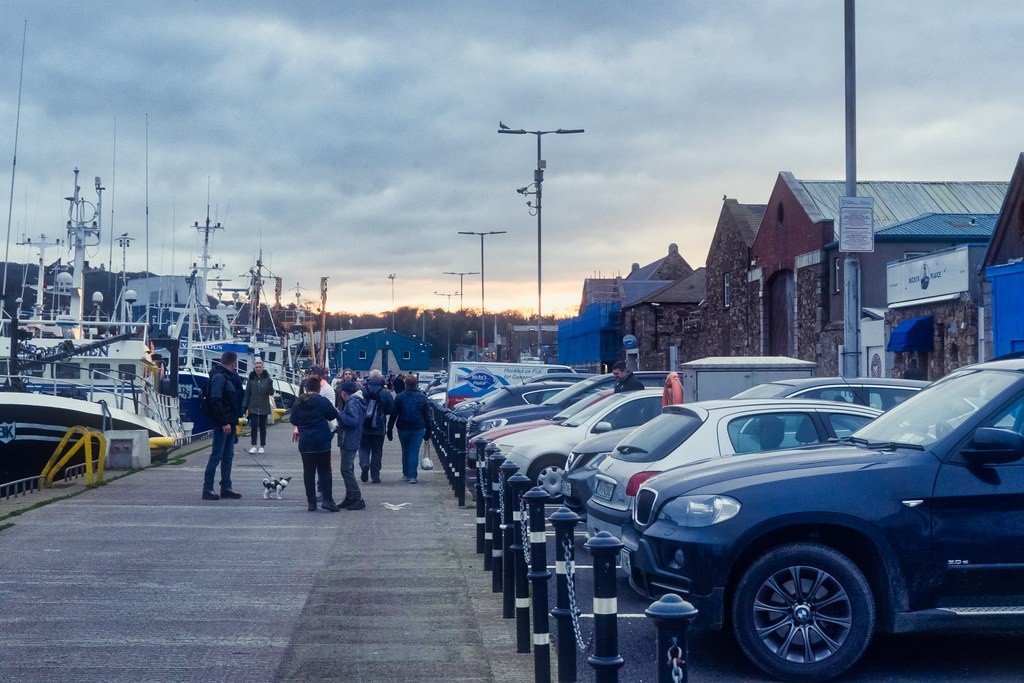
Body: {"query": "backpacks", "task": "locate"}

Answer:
[364,384,384,431]
[199,376,212,416]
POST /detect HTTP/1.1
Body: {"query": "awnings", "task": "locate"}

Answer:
[887,314,934,353]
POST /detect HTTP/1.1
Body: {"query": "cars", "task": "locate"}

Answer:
[421,359,685,506]
[585,396,937,574]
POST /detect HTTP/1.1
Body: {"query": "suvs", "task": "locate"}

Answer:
[620,350,1024,683]
[559,377,1018,515]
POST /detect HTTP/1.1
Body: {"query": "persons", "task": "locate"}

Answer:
[331,368,414,412]
[387,374,433,483]
[359,369,393,483]
[612,361,644,393]
[335,381,367,510]
[242,361,274,453]
[904,359,922,380]
[201,352,241,499]
[289,378,340,512]
[292,365,339,502]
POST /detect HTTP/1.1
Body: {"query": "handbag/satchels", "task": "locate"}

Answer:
[421,457,434,470]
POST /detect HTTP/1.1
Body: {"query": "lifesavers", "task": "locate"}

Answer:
[660,370,684,409]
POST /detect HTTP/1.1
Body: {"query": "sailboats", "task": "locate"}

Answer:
[0,16,338,498]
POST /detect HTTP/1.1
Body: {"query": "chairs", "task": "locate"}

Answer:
[639,397,661,424]
[834,394,848,402]
[761,417,785,451]
[795,415,819,446]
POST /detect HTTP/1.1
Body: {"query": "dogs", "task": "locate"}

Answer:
[263,476,292,500]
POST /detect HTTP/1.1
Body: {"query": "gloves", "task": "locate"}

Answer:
[424,430,431,441]
[387,428,393,441]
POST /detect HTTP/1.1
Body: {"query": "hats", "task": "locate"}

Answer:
[341,381,358,392]
[305,366,322,375]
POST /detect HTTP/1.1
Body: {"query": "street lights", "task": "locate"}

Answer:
[434,292,464,374]
[497,128,587,359]
[457,230,507,362]
[443,271,481,361]
[388,274,397,332]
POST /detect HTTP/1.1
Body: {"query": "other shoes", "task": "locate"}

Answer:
[410,477,416,484]
[258,447,265,454]
[348,499,365,510]
[321,501,340,512]
[309,504,317,511]
[249,447,257,453]
[361,465,369,482]
[338,498,352,508]
[402,474,409,481]
[372,478,381,482]
[316,496,324,502]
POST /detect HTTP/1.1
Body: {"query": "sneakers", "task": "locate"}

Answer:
[202,491,219,500]
[221,490,241,499]
